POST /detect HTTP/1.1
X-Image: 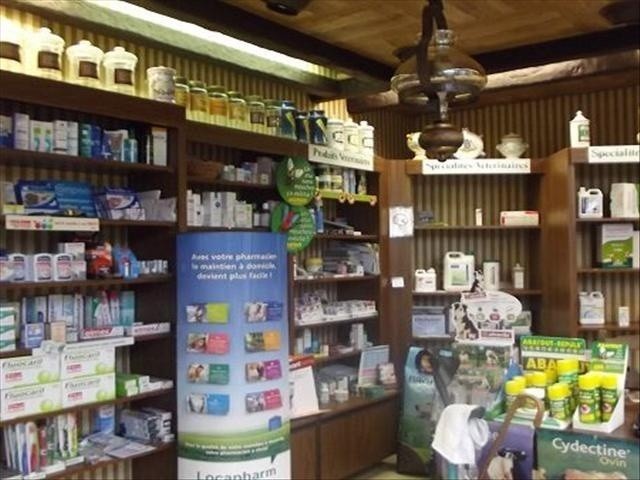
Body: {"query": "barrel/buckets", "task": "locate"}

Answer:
[579,289,605,324]
[444,251,475,290]
[577,187,602,217]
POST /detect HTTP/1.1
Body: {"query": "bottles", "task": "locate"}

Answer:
[1,18,375,191]
[503,357,622,425]
[568,110,592,147]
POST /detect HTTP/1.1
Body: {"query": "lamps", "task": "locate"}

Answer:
[391,0,487,161]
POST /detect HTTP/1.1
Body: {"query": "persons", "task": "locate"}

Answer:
[188,363,204,384]
[186,333,206,352]
